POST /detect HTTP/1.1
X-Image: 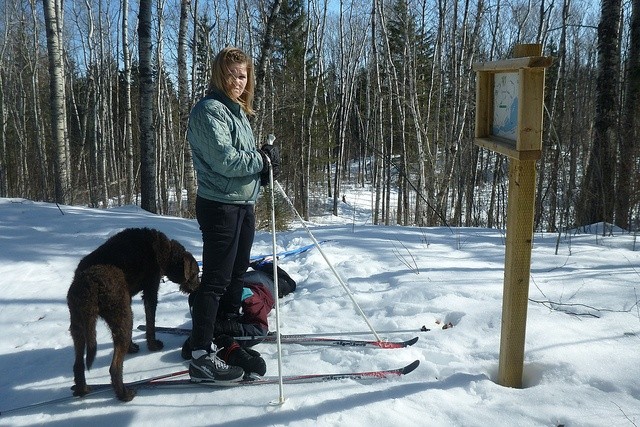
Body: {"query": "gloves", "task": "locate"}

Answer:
[258,143,282,171]
[261,166,283,184]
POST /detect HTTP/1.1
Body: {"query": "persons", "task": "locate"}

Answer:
[187,45,279,387]
[182,258,296,360]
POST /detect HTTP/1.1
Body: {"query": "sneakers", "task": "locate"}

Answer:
[252,256,277,270]
[188,342,245,383]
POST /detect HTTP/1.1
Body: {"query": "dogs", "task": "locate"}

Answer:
[68,226,202,402]
